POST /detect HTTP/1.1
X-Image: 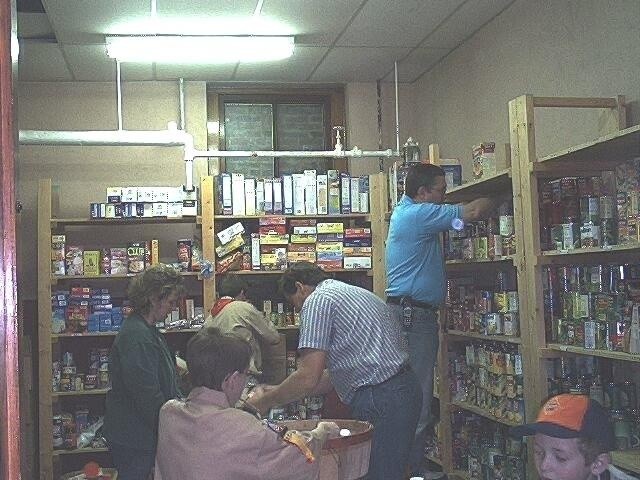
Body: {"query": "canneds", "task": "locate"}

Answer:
[543,264,637,349]
[481,290,519,337]
[544,358,640,450]
[550,195,617,250]
[63,423,77,450]
[53,347,109,392]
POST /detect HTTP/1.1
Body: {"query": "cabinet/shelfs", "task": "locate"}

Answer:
[381,90,640,480]
[382,96,533,479]
[210,169,380,420]
[36,175,209,479]
[517,90,640,478]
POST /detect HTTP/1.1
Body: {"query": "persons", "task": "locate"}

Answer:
[201,273,281,393]
[509,394,638,480]
[106,263,185,480]
[247,260,423,480]
[385,162,497,475]
[155,326,339,479]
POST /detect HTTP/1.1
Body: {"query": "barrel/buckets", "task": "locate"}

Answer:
[274,418,375,479]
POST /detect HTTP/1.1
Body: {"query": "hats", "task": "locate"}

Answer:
[511,394,616,441]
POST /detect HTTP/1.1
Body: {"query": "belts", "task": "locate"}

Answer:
[373,364,411,389]
[387,296,438,312]
